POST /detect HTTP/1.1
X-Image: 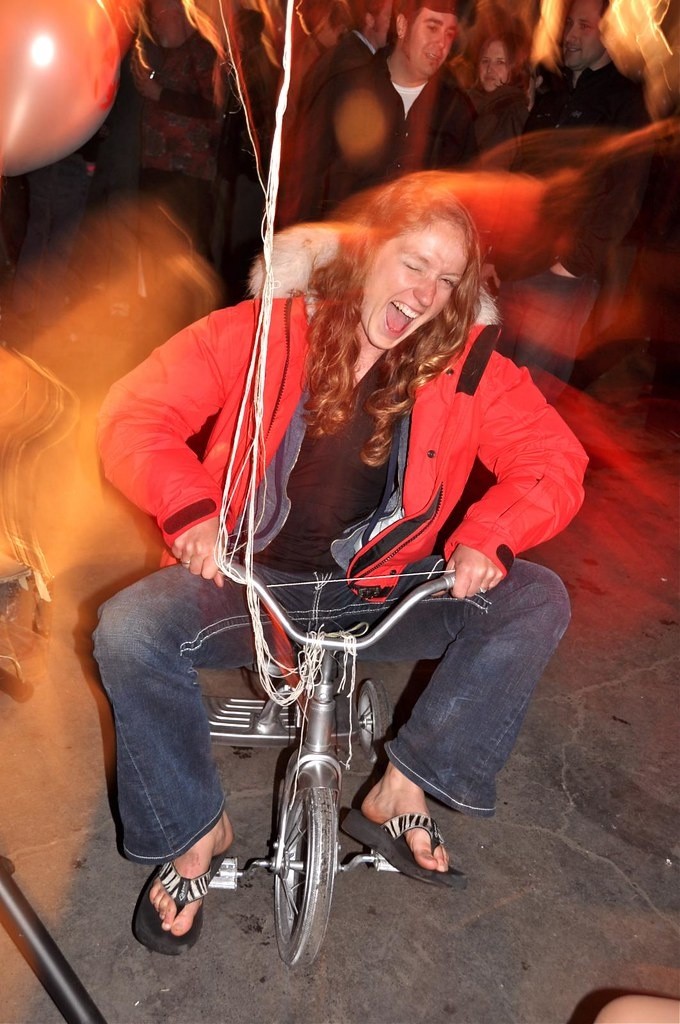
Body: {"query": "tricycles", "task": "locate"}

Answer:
[185,552,457,969]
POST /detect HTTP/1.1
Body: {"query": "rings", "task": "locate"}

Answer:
[480,587,487,593]
[181,560,190,564]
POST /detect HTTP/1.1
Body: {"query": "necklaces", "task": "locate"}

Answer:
[151,10,166,22]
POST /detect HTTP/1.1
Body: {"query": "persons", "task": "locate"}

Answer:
[0,1,680,397]
[93,176,588,956]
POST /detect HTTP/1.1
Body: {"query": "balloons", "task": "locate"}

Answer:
[0,0,121,178]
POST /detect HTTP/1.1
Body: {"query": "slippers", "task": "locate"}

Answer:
[341,808,467,890]
[133,851,227,956]
